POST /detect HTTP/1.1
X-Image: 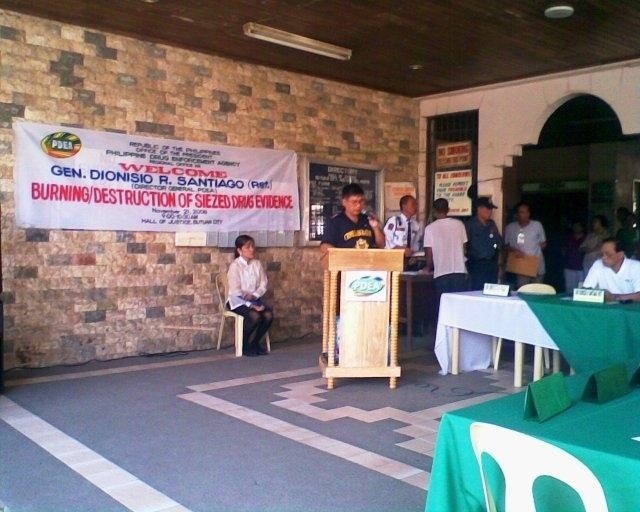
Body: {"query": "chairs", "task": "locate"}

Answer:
[215,272,271,358]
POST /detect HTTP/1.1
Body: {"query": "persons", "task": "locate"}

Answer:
[383,195,426,337]
[464,197,502,290]
[560,207,640,301]
[225,235,273,356]
[422,198,468,292]
[505,204,546,288]
[320,183,385,255]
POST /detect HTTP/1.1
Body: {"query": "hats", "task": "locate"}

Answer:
[475,197,497,209]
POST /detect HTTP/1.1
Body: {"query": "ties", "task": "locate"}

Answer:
[408,222,411,247]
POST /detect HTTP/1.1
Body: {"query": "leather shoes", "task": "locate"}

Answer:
[244,349,265,357]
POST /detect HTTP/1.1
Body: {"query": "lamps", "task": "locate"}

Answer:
[243,22,352,61]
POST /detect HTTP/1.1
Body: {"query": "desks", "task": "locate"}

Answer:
[434,289,640,396]
[399,271,434,351]
[425,355,639,511]
